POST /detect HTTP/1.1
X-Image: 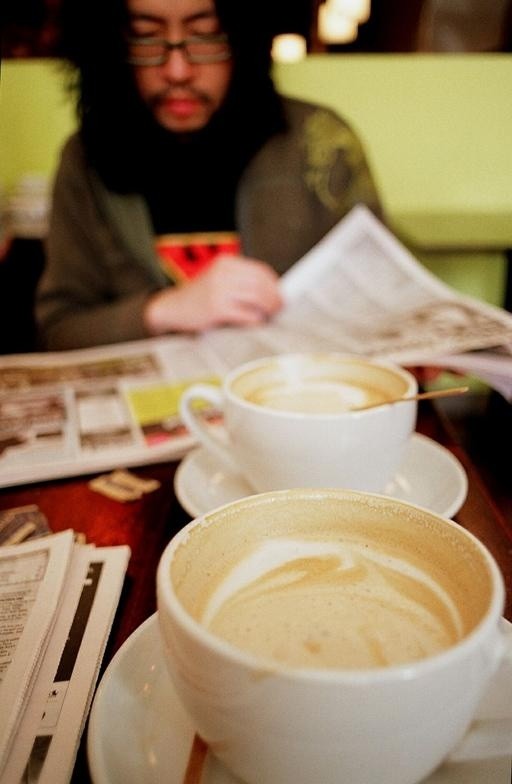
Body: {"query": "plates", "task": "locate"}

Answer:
[173,429,470,523]
[85,608,512,784]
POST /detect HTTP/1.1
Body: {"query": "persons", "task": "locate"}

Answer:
[29,1,386,353]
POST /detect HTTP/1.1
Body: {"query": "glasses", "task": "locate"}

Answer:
[131,35,228,68]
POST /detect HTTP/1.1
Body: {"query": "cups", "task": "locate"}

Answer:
[177,350,419,496]
[154,484,509,783]
